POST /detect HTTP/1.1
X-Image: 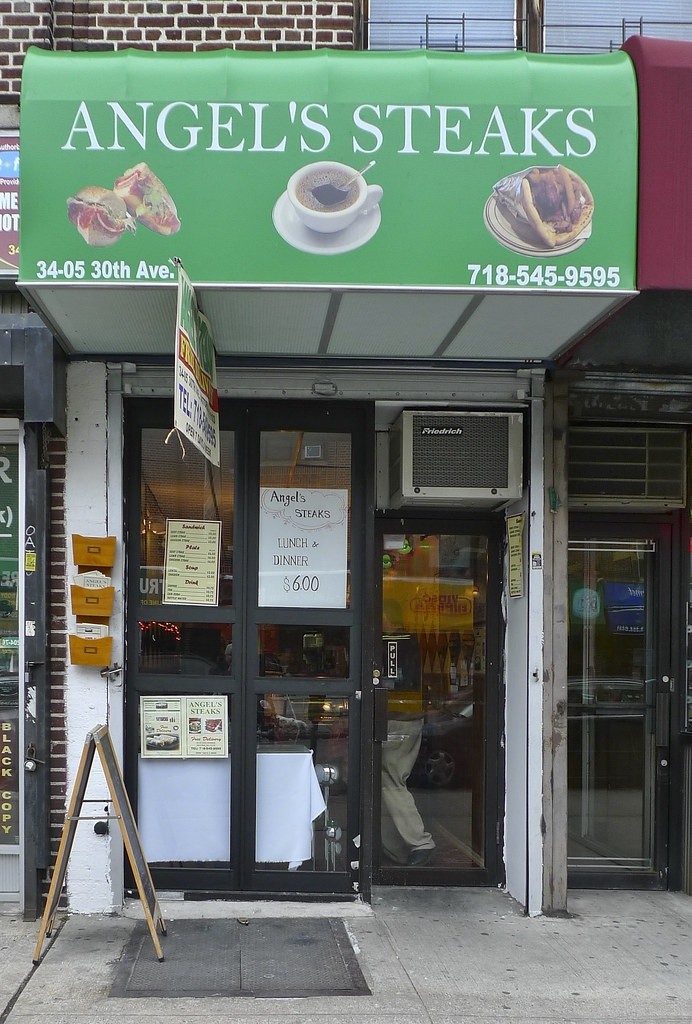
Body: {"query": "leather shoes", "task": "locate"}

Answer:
[409,848,434,866]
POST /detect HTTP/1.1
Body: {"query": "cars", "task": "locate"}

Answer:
[141,651,224,679]
[395,677,648,790]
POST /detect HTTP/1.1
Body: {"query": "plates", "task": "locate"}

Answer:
[482,191,588,257]
[271,189,381,255]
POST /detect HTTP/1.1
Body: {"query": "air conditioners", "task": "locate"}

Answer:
[388,410,524,511]
[304,445,326,461]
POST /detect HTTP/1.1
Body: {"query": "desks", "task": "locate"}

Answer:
[137,743,328,870]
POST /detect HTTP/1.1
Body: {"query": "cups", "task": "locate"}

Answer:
[286,161,384,233]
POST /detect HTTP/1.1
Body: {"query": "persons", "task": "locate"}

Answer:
[382,598,437,865]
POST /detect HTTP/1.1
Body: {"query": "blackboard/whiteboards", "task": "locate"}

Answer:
[44,723,162,928]
